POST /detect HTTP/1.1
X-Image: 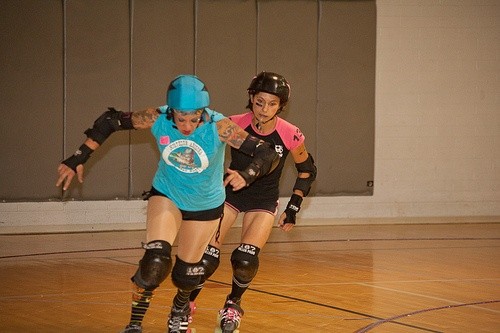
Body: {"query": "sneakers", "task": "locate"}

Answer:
[168,305,189,333]
[187,301,196,327]
[122,324,142,333]
[218,295,244,333]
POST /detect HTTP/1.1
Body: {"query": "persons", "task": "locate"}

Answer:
[55,75,280,333]
[187,71,317,333]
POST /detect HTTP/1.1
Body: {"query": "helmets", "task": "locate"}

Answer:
[166,74,210,112]
[247,72,291,110]
